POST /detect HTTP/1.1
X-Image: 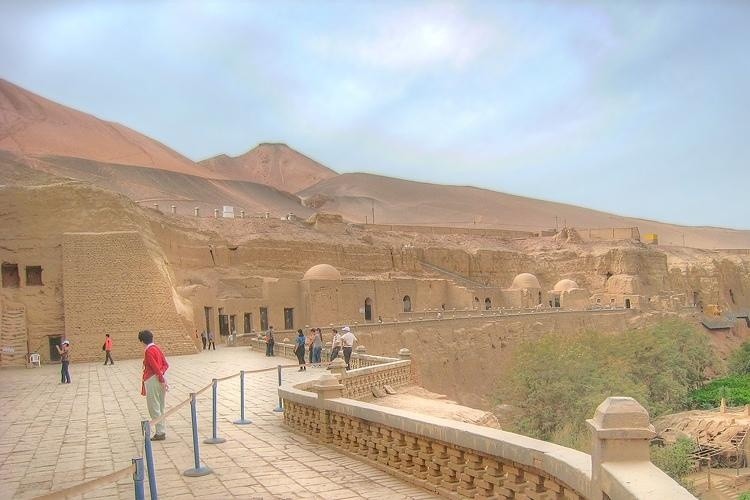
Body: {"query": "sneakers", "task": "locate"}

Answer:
[150,434,165,441]
[297,368,306,372]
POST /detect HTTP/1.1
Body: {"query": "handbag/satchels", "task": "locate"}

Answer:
[141,383,146,396]
[269,339,274,346]
[103,340,109,350]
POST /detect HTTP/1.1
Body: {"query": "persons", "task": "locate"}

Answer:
[265,326,275,356]
[138,329,172,440]
[55,340,72,383]
[103,333,114,365]
[208,330,215,350]
[292,327,359,371]
[200,329,207,350]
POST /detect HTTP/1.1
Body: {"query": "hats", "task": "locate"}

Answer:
[62,341,70,345]
[341,327,351,331]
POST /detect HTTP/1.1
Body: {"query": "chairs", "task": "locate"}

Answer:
[30,354,41,368]
